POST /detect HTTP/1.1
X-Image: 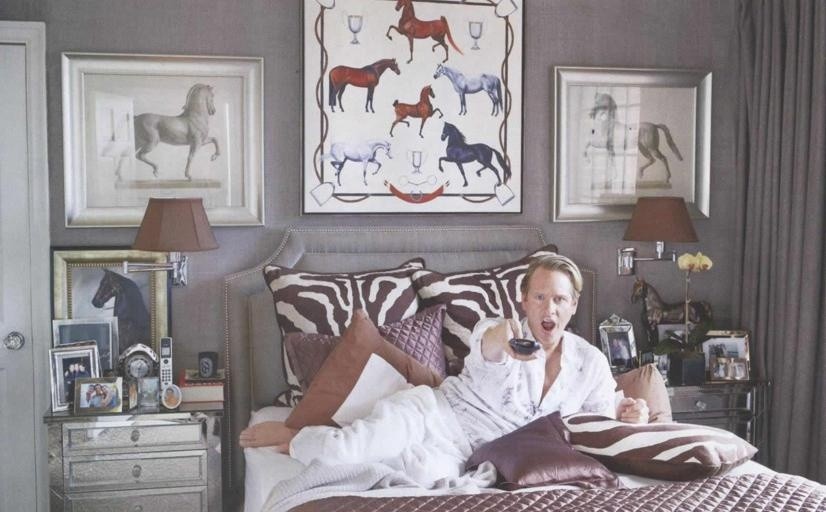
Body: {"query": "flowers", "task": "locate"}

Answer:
[650,252,713,359]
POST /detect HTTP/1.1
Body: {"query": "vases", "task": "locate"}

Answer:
[666,352,705,385]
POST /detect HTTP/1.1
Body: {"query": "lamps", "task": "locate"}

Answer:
[617,197,699,275]
[123,198,219,288]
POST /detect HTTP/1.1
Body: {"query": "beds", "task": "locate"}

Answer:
[223,226,826,512]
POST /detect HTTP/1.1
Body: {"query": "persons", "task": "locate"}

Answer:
[100,385,117,408]
[79,384,95,408]
[237,253,651,470]
[611,335,630,360]
[64,362,91,401]
[708,342,746,378]
[89,384,103,408]
[655,355,670,371]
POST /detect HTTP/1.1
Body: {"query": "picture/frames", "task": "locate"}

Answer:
[598,313,637,373]
[48,345,99,413]
[54,340,103,379]
[74,377,123,415]
[300,0,526,214]
[50,245,172,359]
[552,65,712,224]
[61,52,265,229]
[161,384,182,410]
[52,316,119,373]
[652,322,689,344]
[694,330,755,385]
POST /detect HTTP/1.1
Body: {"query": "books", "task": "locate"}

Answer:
[177,368,226,412]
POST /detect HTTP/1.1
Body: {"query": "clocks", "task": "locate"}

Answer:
[112,344,160,396]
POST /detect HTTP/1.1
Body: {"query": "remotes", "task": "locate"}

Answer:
[508,338,541,355]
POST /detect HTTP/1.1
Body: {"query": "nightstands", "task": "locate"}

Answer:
[42,405,224,512]
[665,378,772,469]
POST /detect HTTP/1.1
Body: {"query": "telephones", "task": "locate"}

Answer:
[159,337,173,397]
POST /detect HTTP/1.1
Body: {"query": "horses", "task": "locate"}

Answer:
[439,121,511,186]
[582,91,684,188]
[433,63,503,116]
[102,83,221,184]
[329,58,400,113]
[318,139,393,186]
[91,267,151,318]
[631,276,713,347]
[390,84,444,138]
[386,0,463,64]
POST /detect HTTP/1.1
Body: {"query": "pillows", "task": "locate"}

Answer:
[285,310,444,431]
[408,244,557,375]
[614,361,672,423]
[465,411,628,492]
[263,257,425,408]
[283,306,446,398]
[562,413,758,481]
